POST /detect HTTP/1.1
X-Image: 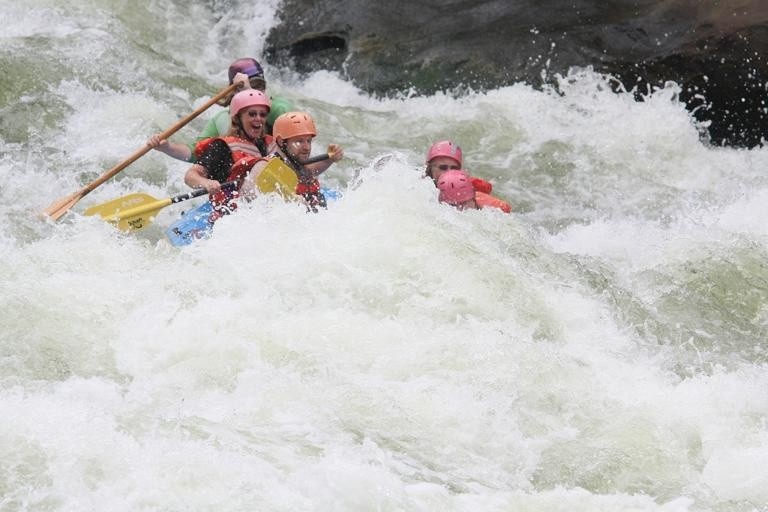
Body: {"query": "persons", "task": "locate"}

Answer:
[425,140,493,194]
[435,169,511,215]
[146,58,344,224]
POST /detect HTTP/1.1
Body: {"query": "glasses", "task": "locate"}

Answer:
[431,164,458,171]
[248,111,266,117]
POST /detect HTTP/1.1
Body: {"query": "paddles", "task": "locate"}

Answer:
[38,82,244,222]
[85,151,336,236]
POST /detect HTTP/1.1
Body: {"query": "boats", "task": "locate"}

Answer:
[163,187,344,248]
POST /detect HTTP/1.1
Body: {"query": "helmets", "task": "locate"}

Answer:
[426,140,462,168]
[230,89,272,123]
[436,170,475,204]
[272,111,317,144]
[228,57,263,85]
